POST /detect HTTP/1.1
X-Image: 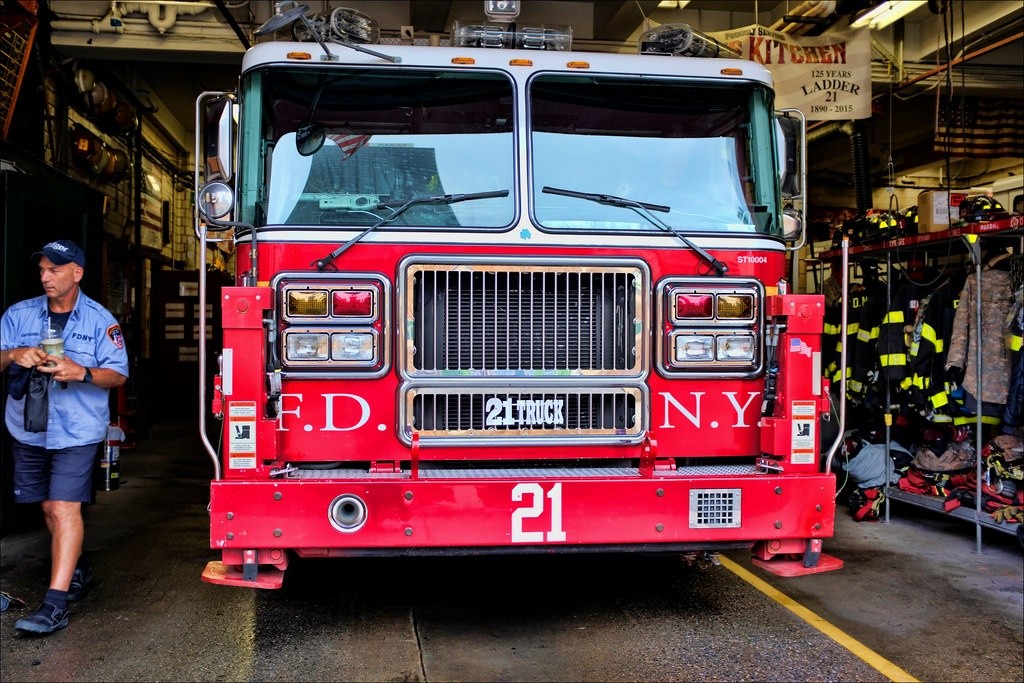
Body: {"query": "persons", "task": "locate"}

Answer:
[0,239,128,636]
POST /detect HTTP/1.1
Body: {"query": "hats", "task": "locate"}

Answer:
[31,239,86,268]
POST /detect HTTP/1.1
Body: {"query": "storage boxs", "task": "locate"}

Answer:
[916,189,969,233]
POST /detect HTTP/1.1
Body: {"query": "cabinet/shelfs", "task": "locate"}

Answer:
[807,220,1024,551]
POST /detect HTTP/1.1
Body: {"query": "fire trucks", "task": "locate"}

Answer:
[192,5,844,591]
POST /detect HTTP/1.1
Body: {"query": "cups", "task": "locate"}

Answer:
[38,339,65,367]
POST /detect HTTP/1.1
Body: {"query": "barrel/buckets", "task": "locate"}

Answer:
[95,439,121,491]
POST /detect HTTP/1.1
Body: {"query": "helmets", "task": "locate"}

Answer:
[866,211,902,242]
[901,207,922,235]
[961,196,1006,223]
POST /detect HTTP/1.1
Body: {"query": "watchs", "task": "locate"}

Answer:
[78,367,92,385]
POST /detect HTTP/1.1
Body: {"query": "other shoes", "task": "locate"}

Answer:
[15,603,70,633]
[66,568,87,603]
